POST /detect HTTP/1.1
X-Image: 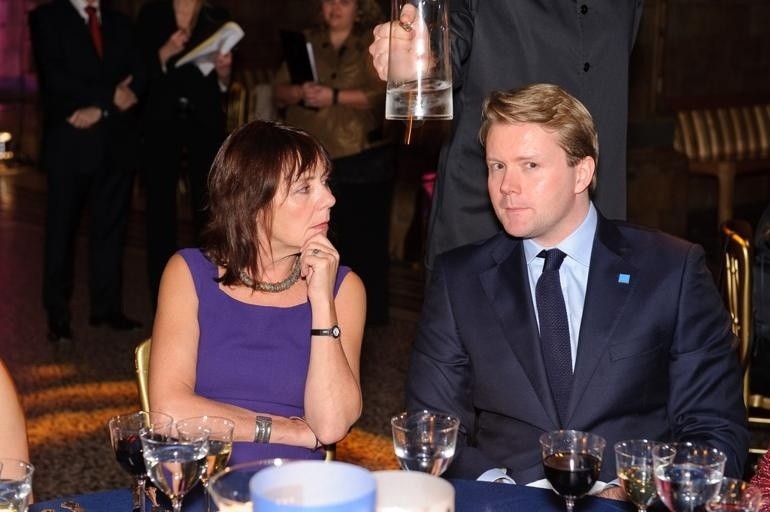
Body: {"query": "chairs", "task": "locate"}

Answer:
[718,218,770,454]
[135,338,154,429]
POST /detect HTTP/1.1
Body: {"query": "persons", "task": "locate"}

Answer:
[369,2,641,284]
[146,118,367,486]
[402,83,750,511]
[355,3,449,324]
[273,2,386,265]
[27,1,233,340]
[0,359,36,507]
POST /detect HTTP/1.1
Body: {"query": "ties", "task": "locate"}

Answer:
[536,248,573,429]
[84,5,103,60]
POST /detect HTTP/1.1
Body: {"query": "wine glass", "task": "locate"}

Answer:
[538,429,606,512]
[108,409,235,512]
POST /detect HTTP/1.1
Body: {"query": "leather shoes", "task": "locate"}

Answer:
[46,318,71,343]
[89,312,143,331]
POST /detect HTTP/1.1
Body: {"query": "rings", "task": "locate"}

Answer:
[312,249,320,256]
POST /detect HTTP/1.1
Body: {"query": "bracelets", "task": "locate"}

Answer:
[289,416,318,452]
[333,89,339,104]
[254,416,273,444]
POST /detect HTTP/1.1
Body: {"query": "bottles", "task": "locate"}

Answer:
[384,0,456,124]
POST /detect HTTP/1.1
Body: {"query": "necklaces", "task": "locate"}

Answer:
[240,254,301,292]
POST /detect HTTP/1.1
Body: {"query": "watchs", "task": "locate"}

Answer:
[310,325,341,338]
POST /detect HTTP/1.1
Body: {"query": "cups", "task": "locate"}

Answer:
[613,440,764,512]
[209,457,457,511]
[1,455,34,512]
[391,408,461,476]
